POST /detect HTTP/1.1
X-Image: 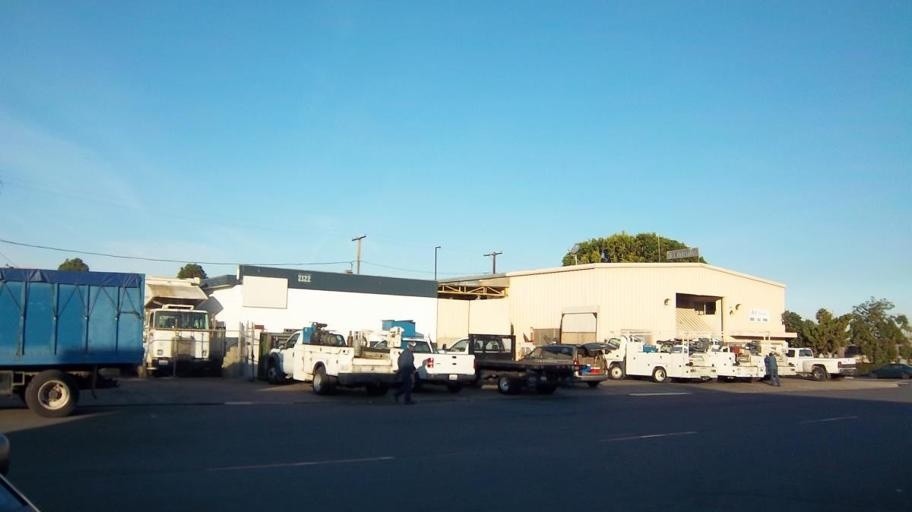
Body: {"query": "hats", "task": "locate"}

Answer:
[407,341,417,347]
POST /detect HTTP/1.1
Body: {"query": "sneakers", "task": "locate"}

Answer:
[405,399,416,404]
[393,392,399,402]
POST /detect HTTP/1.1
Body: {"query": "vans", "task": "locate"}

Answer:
[516,343,609,387]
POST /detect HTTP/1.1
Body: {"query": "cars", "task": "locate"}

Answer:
[868,363,912,380]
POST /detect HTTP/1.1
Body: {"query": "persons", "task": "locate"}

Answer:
[392,339,420,405]
[765,353,781,387]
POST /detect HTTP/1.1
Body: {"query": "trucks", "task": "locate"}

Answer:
[441,333,588,396]
[134,277,212,379]
[263,322,402,396]
[603,334,857,383]
[1,265,145,420]
[360,330,477,392]
